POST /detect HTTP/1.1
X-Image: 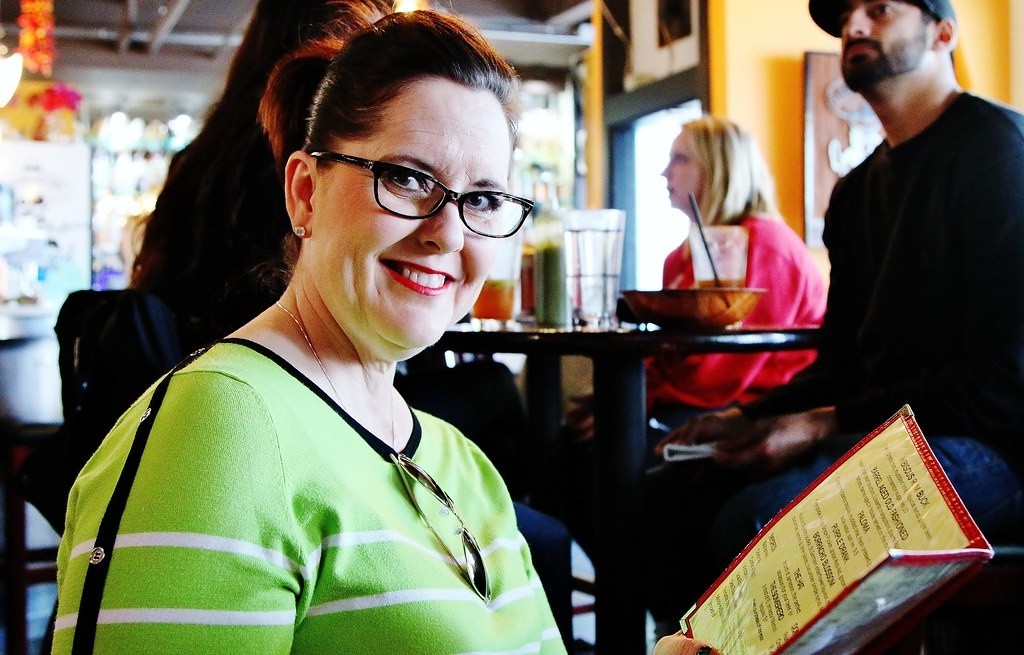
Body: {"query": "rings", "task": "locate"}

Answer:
[696,645,711,655]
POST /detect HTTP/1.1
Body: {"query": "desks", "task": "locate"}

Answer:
[434,318,823,655]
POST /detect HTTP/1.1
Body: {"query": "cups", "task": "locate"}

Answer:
[562,208,626,332]
[533,208,572,329]
[469,232,519,332]
[688,224,750,333]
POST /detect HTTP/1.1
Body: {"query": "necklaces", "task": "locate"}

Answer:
[275,301,397,452]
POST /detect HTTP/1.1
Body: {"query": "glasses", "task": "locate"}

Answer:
[390,454,491,604]
[308,150,535,238]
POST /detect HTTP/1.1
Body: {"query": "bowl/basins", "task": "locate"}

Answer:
[618,286,767,332]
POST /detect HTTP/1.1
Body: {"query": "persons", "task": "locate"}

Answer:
[49,0,568,655]
[530,1,1023,566]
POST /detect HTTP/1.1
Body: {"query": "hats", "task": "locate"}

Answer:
[808,0,955,38]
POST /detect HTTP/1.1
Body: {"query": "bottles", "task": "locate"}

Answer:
[515,163,568,324]
